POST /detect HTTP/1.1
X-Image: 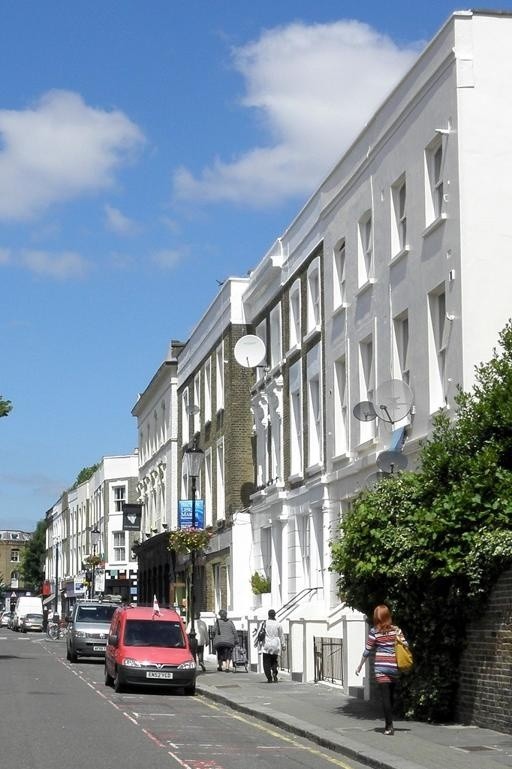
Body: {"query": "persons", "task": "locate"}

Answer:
[253,609,287,683]
[211,609,238,672]
[355,603,409,734]
[185,612,210,671]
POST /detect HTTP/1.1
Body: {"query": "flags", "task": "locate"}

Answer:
[153,595,161,614]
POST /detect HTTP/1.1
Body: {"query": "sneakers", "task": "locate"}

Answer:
[267,674,278,683]
[202,666,229,673]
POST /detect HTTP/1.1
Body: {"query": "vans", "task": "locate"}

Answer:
[65,599,197,693]
[11,597,44,631]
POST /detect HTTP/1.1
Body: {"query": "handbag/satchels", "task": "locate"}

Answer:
[395,629,415,674]
[257,620,267,641]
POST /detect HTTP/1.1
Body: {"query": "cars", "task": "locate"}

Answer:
[0,612,11,628]
[21,613,44,633]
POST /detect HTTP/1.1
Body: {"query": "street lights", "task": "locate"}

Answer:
[91,526,100,598]
[52,535,61,612]
[186,438,204,667]
